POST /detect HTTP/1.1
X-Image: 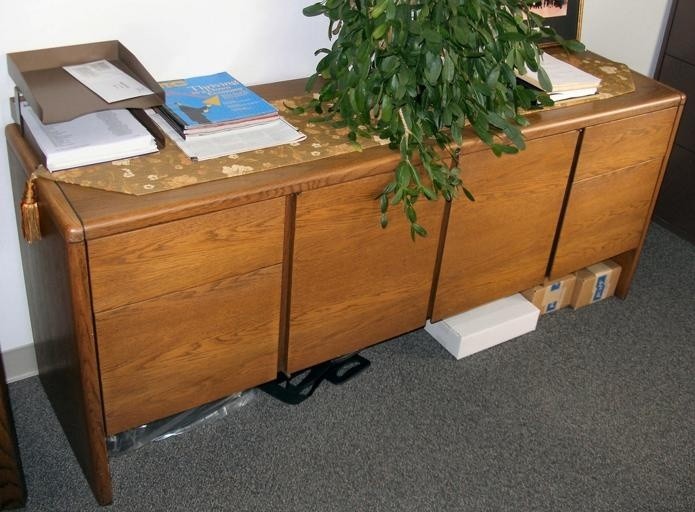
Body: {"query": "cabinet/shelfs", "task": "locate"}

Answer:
[651,1,695,246]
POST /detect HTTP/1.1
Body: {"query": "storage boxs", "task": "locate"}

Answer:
[425,291,541,360]
[570,259,622,310]
[520,273,577,315]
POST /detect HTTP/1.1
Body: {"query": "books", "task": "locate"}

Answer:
[152,72,280,141]
[513,52,601,103]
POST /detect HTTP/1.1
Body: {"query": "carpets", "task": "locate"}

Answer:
[1,219,695,512]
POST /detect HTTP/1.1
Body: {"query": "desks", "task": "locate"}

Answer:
[5,44,687,506]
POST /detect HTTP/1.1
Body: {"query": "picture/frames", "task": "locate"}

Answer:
[500,0,585,43]
